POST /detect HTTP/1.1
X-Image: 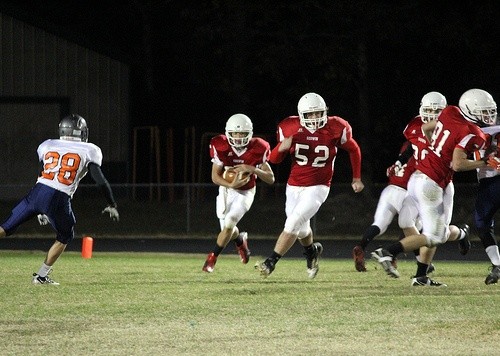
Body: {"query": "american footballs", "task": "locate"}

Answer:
[225,168,255,191]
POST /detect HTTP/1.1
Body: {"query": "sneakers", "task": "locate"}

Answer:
[202,251,218,273]
[412,276,447,288]
[370,247,401,278]
[353,244,367,272]
[410,262,436,279]
[485,263,500,285]
[303,242,323,279]
[457,224,471,256]
[235,231,251,264]
[32,273,60,286]
[254,256,275,280]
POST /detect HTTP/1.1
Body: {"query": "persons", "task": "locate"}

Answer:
[353,89,500,289]
[0,114,119,286]
[254,94,365,280]
[202,114,275,272]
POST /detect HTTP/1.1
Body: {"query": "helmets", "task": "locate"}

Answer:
[58,114,88,143]
[458,88,497,127]
[419,91,448,124]
[297,92,328,130]
[225,113,254,148]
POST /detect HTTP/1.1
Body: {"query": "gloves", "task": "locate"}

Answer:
[386,164,400,178]
[103,206,120,224]
[37,213,51,226]
